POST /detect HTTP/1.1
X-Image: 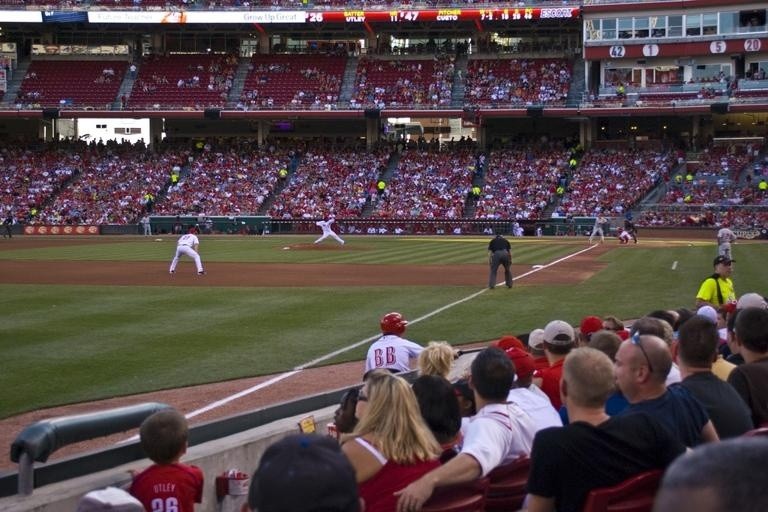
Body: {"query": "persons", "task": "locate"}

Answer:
[169,226,208,277]
[313,217,345,247]
[2,216,14,240]
[616,226,630,244]
[141,213,153,240]
[694,255,737,309]
[622,219,638,244]
[3,1,768,220]
[587,214,608,247]
[485,233,513,289]
[345,222,592,236]
[716,221,738,266]
[174,217,274,235]
[240,433,367,511]
[331,293,768,512]
[126,409,204,511]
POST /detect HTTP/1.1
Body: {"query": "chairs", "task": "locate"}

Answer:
[420,479,488,512]
[0,90,4,97]
[26,0,60,5]
[735,90,768,97]
[737,427,768,439]
[485,457,531,512]
[586,470,664,512]
[94,0,183,6]
[315,0,412,6]
[639,94,697,106]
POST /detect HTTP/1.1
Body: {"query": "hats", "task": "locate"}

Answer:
[528,329,544,351]
[545,320,575,345]
[714,255,736,265]
[495,336,524,351]
[581,316,603,335]
[505,347,536,379]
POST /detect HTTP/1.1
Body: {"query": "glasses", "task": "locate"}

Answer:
[632,330,653,374]
[357,390,367,402]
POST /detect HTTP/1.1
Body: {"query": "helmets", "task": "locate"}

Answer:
[380,312,407,334]
